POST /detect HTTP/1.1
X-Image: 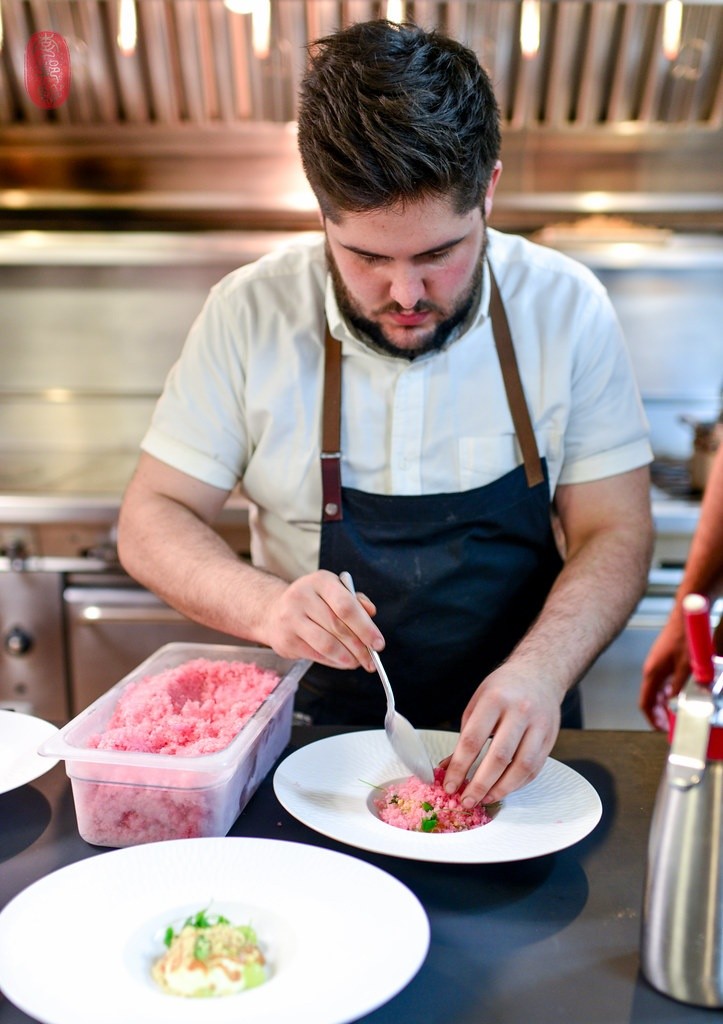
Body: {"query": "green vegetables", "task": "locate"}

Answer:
[163,907,228,964]
[417,801,473,833]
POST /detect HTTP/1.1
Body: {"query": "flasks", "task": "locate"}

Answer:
[643,600,723,1008]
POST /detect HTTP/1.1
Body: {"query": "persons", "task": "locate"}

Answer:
[639,414,723,734]
[116,18,654,806]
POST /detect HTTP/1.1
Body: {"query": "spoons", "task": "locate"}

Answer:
[336,569,436,789]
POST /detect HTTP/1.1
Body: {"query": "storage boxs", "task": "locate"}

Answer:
[34,642,315,847]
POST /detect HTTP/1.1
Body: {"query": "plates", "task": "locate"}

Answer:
[0,836,433,1024]
[273,727,602,863]
[0,710,65,796]
